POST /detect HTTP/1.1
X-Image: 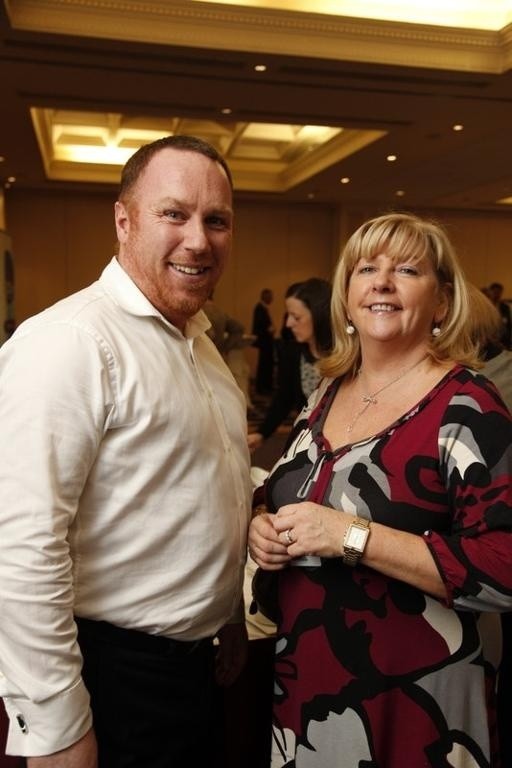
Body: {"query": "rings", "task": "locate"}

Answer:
[284,528,295,545]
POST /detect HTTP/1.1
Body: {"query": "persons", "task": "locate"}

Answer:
[240,208,512,767]
[247,277,336,454]
[471,283,512,409]
[1,135,274,767]
[252,288,274,396]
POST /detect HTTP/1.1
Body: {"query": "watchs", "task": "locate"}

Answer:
[340,517,372,567]
